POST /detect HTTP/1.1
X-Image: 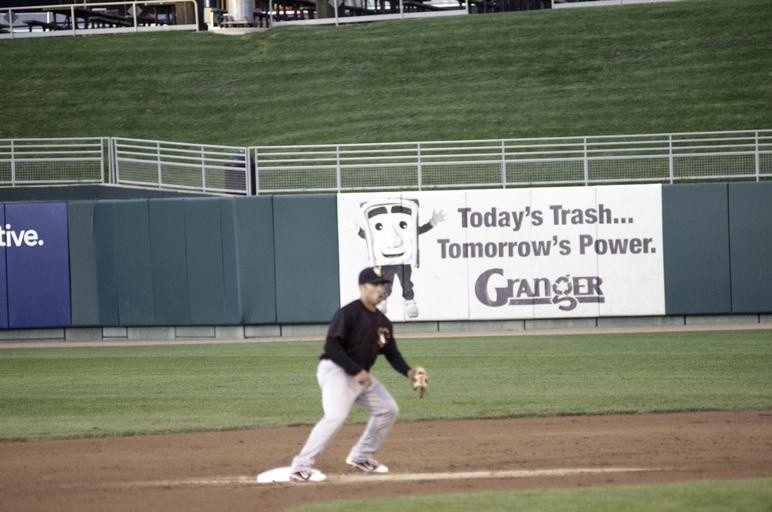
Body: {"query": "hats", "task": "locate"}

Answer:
[359,268,392,284]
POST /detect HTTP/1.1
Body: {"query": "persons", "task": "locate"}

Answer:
[289,266,425,482]
[354,196,447,318]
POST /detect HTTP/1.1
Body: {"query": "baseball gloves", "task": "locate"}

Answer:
[410,366,429,398]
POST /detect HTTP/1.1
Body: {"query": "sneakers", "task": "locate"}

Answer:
[345,456,390,474]
[289,468,327,482]
